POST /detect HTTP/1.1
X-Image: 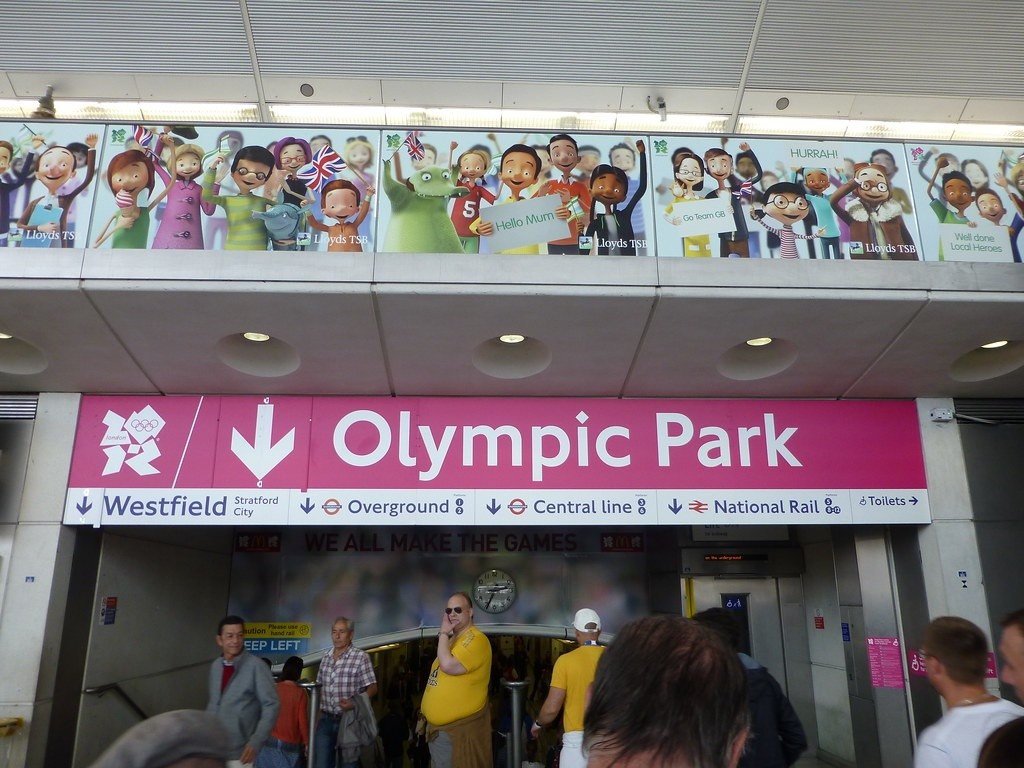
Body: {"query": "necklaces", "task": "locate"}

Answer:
[956,693,989,704]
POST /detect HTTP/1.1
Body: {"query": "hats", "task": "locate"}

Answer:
[88,709,229,768]
[284,656,306,669]
[571,608,601,633]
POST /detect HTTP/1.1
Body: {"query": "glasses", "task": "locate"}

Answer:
[444,607,470,614]
[917,648,943,665]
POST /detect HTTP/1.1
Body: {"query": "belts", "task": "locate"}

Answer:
[320,709,342,718]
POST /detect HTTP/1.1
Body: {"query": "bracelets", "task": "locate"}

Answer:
[535,720,541,728]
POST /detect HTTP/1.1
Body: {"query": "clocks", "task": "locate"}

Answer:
[472,567,518,615]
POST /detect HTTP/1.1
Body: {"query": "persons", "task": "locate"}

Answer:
[91,709,233,768]
[205,616,280,768]
[915,616,1024,768]
[976,611,1024,768]
[316,618,377,768]
[269,656,310,768]
[421,592,493,768]
[377,694,430,768]
[692,608,808,768]
[530,608,607,768]
[583,616,751,768]
